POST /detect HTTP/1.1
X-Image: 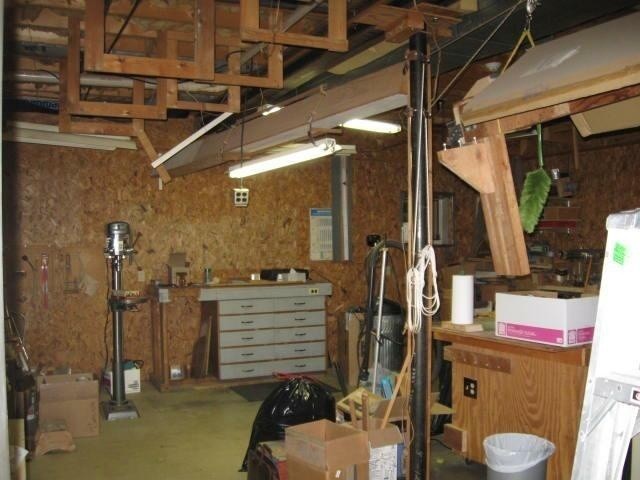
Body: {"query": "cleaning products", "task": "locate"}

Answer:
[202,242,212,285]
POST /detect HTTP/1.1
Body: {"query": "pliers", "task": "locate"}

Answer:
[40,254,48,311]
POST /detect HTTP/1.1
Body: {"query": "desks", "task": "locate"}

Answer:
[430,324,591,479]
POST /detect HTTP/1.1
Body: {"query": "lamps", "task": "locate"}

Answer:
[223,100,403,181]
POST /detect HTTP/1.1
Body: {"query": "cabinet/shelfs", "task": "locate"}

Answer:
[146,277,333,394]
[535,182,586,230]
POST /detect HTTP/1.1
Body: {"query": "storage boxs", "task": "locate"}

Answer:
[493,290,599,347]
[104,365,140,397]
[284,387,456,480]
[34,371,101,439]
[334,302,367,397]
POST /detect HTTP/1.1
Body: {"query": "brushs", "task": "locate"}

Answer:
[63,254,80,294]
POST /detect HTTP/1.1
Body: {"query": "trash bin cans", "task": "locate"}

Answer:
[483,433,548,480]
[371,299,406,374]
